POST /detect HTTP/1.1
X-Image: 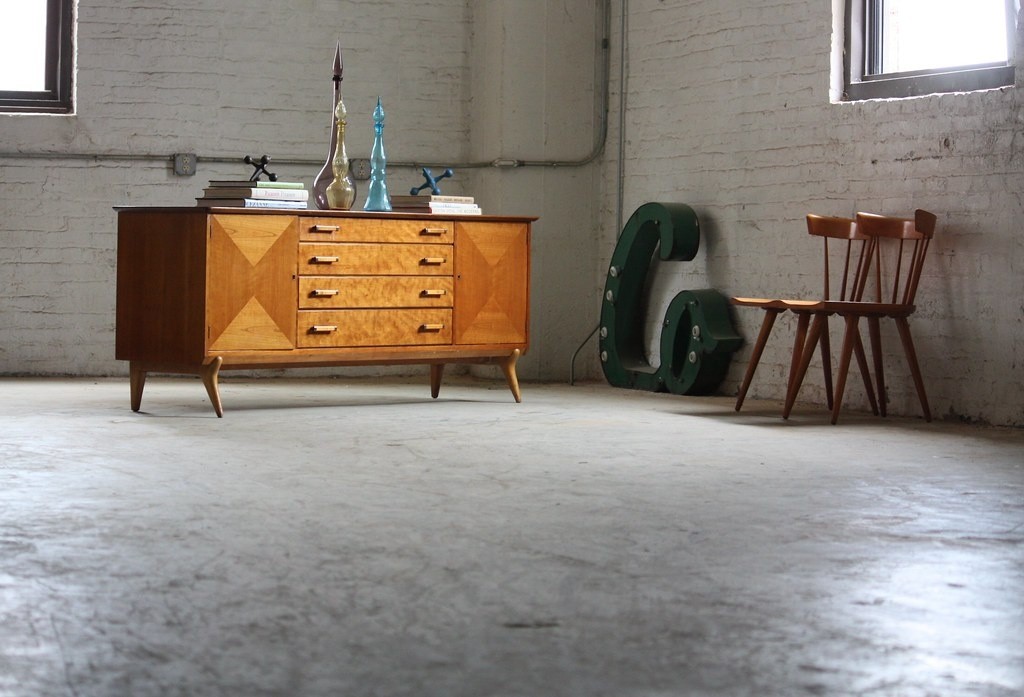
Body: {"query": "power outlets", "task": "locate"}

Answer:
[349,158,371,180]
[173,153,197,176]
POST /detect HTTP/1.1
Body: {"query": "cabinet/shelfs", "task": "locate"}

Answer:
[112,206,539,418]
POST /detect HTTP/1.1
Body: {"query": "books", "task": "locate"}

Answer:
[195,179,309,209]
[390,195,482,215]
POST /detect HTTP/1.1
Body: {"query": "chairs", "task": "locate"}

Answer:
[729,213,880,416]
[783,209,937,426]
